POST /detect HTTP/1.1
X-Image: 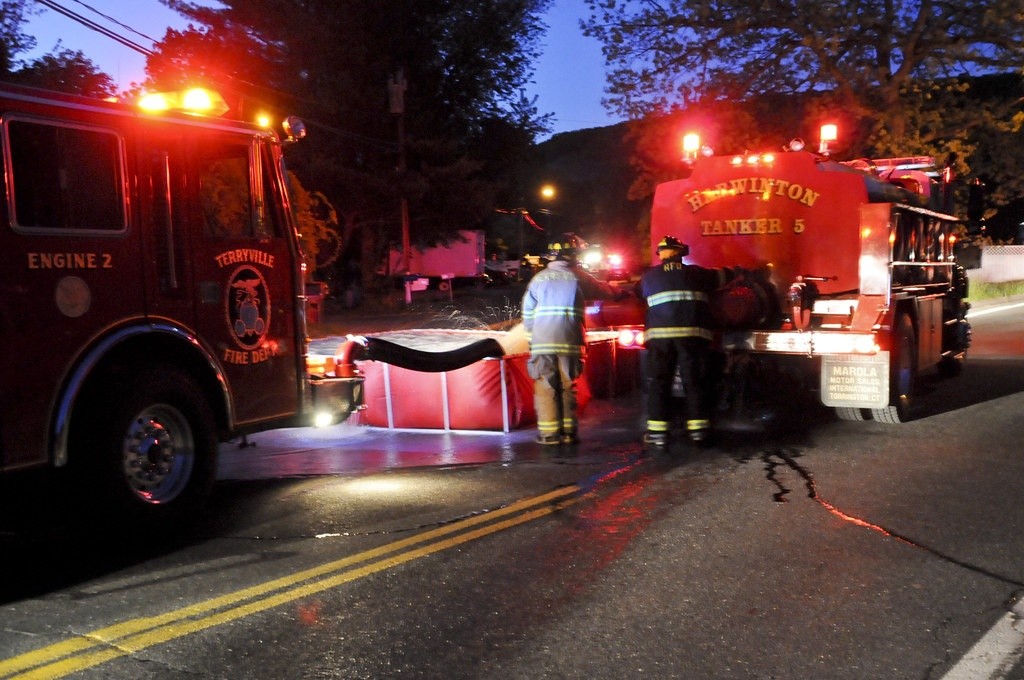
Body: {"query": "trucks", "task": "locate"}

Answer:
[385,227,485,292]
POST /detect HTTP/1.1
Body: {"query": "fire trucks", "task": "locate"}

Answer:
[0,72,312,555]
[645,124,974,426]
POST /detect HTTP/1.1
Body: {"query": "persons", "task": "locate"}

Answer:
[521,236,634,444]
[632,237,746,445]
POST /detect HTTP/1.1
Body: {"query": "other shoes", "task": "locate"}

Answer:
[535,434,573,445]
[687,429,701,441]
[643,432,664,451]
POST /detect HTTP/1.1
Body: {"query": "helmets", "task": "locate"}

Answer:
[544,233,587,255]
[656,235,690,256]
